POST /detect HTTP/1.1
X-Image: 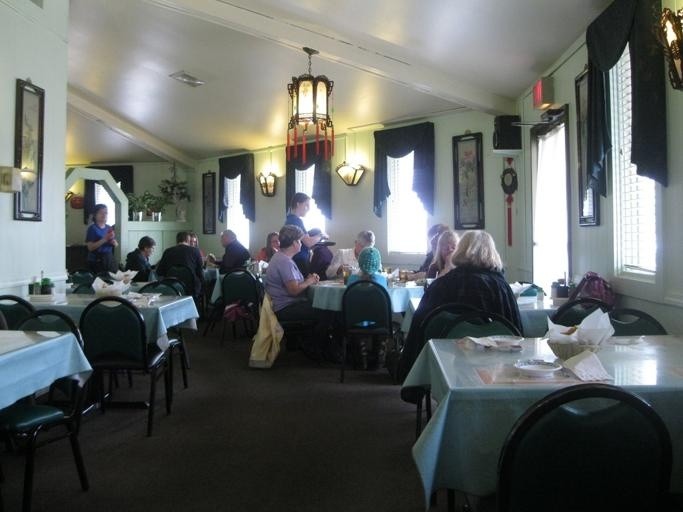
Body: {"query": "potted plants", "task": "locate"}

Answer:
[127,192,146,222]
[143,192,166,222]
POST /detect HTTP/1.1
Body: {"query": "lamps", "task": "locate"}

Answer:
[256,166,277,198]
[650,6,682,91]
[335,161,363,186]
[67,192,84,209]
[283,46,335,164]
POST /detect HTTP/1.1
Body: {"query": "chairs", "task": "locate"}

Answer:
[516,280,545,296]
[246,274,320,373]
[338,280,399,383]
[0,295,40,328]
[492,385,673,512]
[608,307,666,337]
[139,282,188,389]
[73,270,184,296]
[542,298,609,335]
[408,301,486,443]
[0,310,90,512]
[442,310,523,338]
[77,297,171,438]
[219,271,258,342]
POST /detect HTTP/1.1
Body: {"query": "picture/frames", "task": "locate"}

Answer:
[202,171,216,235]
[572,69,599,227]
[451,133,484,230]
[12,78,45,222]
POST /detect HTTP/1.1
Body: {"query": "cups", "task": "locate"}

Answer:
[398,268,406,281]
[342,266,352,284]
[384,266,391,274]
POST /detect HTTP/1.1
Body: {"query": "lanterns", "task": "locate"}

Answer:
[284,74,339,166]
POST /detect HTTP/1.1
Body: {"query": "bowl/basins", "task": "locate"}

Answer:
[547,339,601,359]
[93,288,121,298]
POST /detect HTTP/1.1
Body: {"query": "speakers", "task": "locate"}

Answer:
[493,115,521,149]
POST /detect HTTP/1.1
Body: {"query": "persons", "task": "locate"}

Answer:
[253,230,280,264]
[158,231,205,286]
[302,228,334,275]
[125,235,156,281]
[279,192,330,277]
[212,228,249,279]
[424,229,460,280]
[398,228,526,379]
[399,222,451,282]
[187,231,217,268]
[262,224,343,359]
[83,203,119,268]
[346,229,390,372]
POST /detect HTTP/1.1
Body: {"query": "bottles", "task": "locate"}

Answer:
[32,278,51,296]
[537,287,544,300]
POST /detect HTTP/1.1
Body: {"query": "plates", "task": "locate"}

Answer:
[514,359,562,376]
[488,334,523,346]
